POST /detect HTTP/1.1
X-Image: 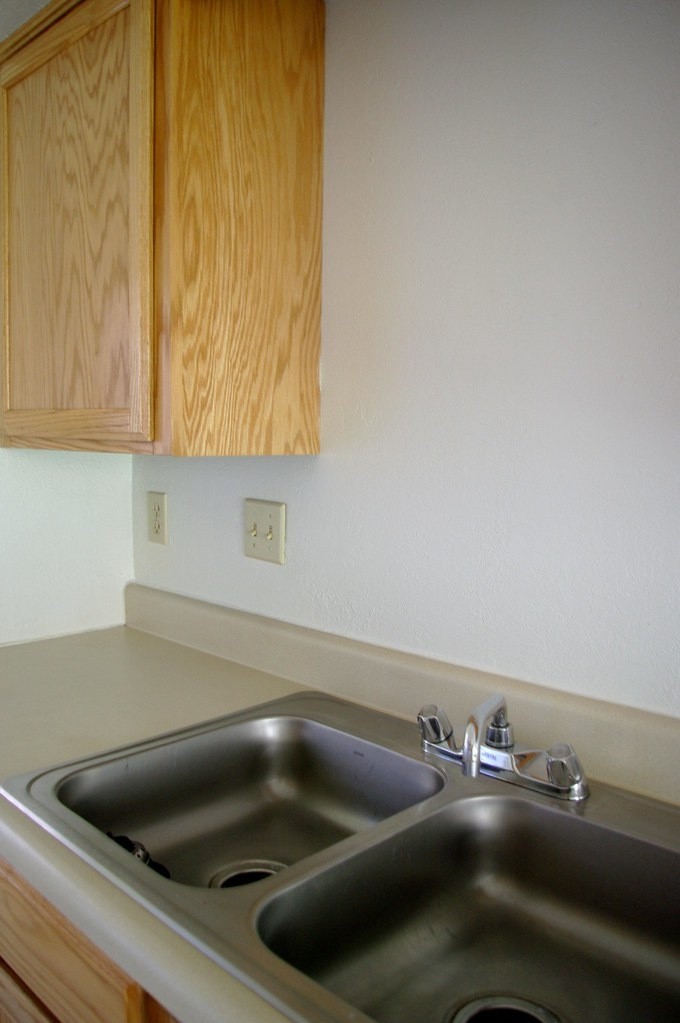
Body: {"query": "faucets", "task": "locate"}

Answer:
[462,692,514,780]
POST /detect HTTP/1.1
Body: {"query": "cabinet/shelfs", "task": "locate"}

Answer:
[0,1,329,457]
[0,857,180,1023]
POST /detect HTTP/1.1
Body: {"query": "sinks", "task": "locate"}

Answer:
[252,786,680,1023]
[50,711,453,895]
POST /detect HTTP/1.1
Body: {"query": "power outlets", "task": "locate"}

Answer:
[240,501,286,563]
[146,491,168,544]
